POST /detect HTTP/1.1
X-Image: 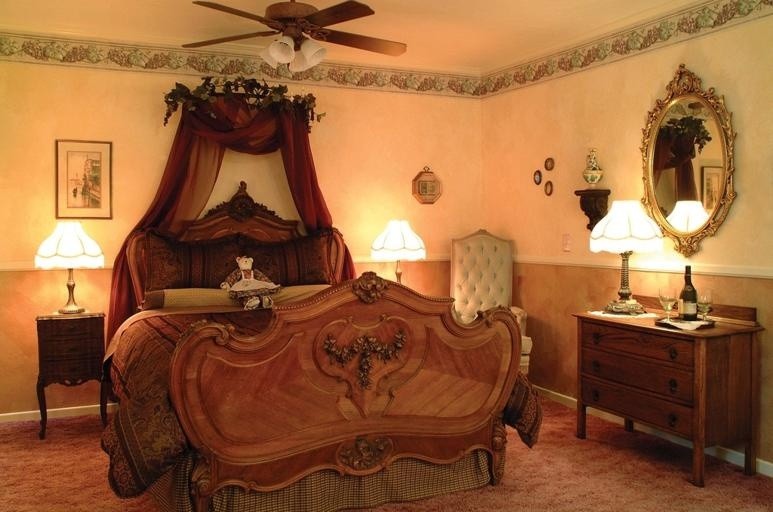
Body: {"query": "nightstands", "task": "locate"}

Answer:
[35,312,111,440]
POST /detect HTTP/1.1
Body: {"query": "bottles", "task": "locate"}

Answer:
[679,265,697,321]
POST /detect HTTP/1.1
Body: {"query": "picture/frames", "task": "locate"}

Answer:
[701,166,725,209]
[56,139,112,219]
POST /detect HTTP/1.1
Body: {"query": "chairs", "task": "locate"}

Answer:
[450,228,533,374]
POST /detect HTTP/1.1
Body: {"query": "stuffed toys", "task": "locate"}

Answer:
[220,256,276,310]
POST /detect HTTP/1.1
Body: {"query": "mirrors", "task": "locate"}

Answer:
[639,63,737,257]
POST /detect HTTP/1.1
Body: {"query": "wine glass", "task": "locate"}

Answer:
[697,288,713,324]
[659,286,678,322]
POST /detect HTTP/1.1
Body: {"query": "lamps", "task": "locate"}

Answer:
[261,26,326,72]
[371,219,426,284]
[590,201,664,314]
[34,221,105,314]
[666,200,709,234]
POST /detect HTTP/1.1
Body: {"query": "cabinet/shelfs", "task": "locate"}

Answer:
[571,306,765,487]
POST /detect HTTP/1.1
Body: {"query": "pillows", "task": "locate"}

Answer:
[142,284,334,308]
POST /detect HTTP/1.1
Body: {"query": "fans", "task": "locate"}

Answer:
[181,0,407,57]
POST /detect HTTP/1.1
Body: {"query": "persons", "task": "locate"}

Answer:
[82,173,90,207]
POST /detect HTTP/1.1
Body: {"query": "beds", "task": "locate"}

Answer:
[101,181,543,511]
